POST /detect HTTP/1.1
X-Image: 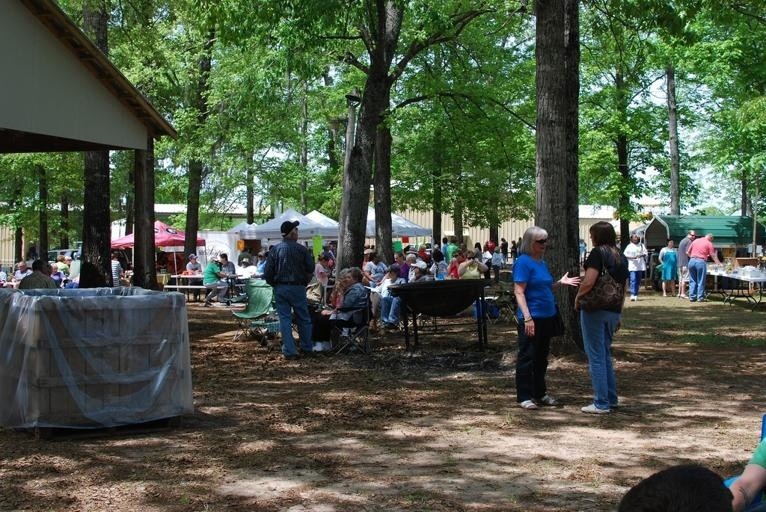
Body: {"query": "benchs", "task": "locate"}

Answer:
[164,284,225,303]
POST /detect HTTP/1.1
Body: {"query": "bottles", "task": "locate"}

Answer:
[725,258,732,274]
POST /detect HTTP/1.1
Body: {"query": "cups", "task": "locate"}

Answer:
[714,265,718,273]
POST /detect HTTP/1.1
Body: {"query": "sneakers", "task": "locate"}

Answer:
[520,400,537,409]
[581,404,611,414]
[629,278,724,315]
[258,312,421,371]
[531,395,559,406]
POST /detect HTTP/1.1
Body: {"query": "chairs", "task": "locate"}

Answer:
[483,272,517,324]
[231,275,369,356]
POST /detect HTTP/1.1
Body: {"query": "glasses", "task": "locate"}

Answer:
[468,257,475,260]
[536,239,547,244]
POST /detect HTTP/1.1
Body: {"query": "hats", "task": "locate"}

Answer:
[186,234,278,269]
[281,221,299,237]
[411,260,427,270]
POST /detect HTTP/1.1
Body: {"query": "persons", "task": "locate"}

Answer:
[429,234,523,284]
[685,233,724,303]
[574,222,631,415]
[510,226,581,411]
[623,233,649,303]
[217,251,236,306]
[657,237,680,298]
[0,252,124,291]
[729,437,765,511]
[618,464,734,511]
[676,229,697,300]
[185,253,204,301]
[579,239,587,264]
[233,240,434,353]
[198,255,230,307]
[264,221,315,358]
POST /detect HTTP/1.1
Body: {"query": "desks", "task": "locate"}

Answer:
[705,265,766,312]
[170,272,230,301]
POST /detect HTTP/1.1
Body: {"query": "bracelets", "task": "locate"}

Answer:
[523,317,534,323]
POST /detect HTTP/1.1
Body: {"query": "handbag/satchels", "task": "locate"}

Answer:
[549,305,564,337]
[576,269,626,313]
